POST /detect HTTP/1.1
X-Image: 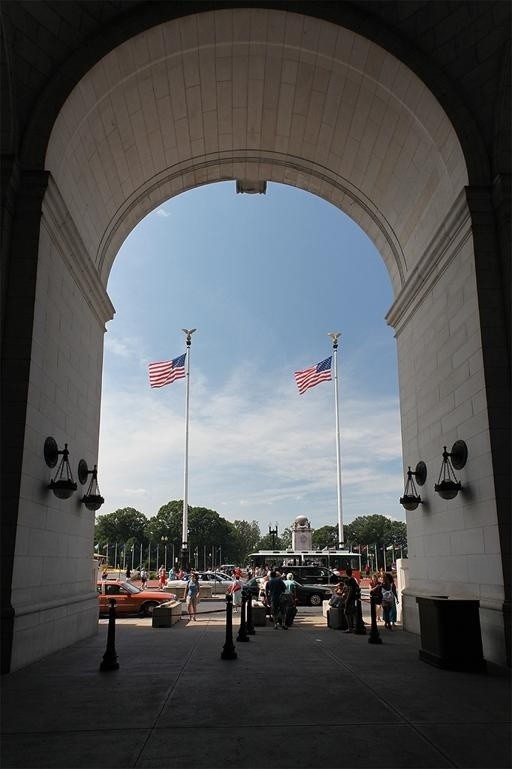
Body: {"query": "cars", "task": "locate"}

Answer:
[165,564,352,605]
[95,579,177,616]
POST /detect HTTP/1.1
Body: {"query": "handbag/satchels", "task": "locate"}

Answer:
[278,592,294,607]
[233,582,241,591]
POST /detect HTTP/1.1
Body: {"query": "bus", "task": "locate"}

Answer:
[247,547,362,584]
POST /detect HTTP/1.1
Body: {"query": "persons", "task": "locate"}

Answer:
[140,567,147,590]
[104,568,108,578]
[96,587,101,595]
[145,569,149,586]
[106,585,114,594]
[101,572,107,582]
[158,563,399,634]
[125,566,131,582]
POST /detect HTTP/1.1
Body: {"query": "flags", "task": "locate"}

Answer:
[294,354,333,396]
[148,352,187,389]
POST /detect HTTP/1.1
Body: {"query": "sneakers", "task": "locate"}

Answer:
[282,625,289,630]
[272,626,279,631]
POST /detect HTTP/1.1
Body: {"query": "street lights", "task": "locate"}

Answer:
[267,521,279,550]
[160,535,168,571]
[194,549,199,568]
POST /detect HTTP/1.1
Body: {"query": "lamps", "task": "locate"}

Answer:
[78,459,103,510]
[44,437,78,498]
[433,439,468,499]
[400,460,427,511]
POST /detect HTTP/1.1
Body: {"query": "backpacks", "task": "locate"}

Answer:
[380,583,395,608]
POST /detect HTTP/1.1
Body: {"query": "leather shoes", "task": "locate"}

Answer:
[341,628,352,633]
